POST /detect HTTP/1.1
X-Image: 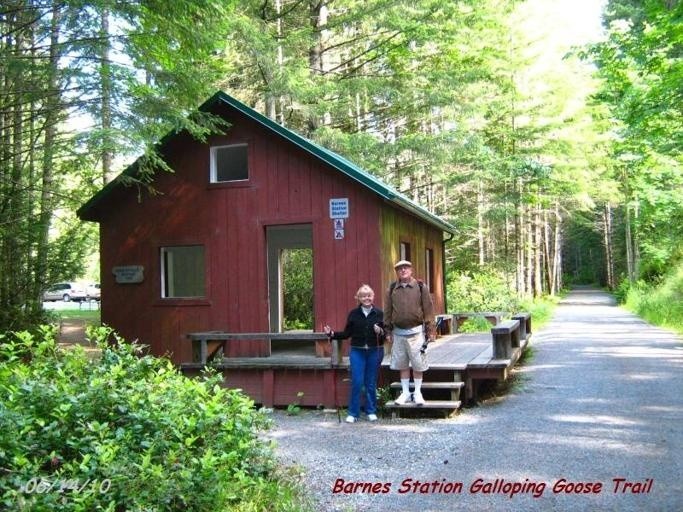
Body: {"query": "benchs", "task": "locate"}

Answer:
[435,315,452,337]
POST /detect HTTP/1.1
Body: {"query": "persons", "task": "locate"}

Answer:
[384,260,436,405]
[324,285,386,423]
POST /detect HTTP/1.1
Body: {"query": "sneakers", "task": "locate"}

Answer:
[413,392,425,405]
[367,414,378,422]
[345,415,359,423]
[394,392,412,405]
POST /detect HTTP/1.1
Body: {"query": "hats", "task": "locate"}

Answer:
[394,259,413,268]
